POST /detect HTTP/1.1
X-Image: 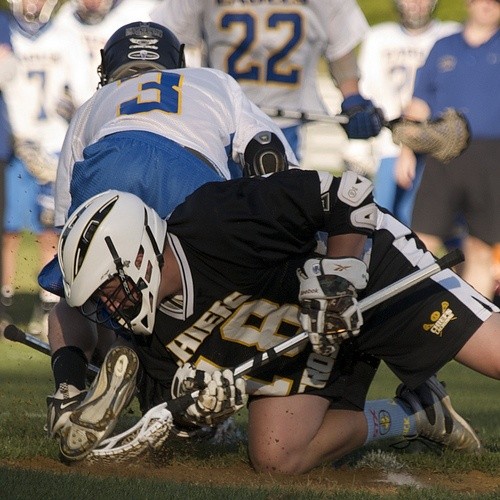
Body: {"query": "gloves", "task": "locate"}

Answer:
[341,94,383,140]
[295,253,368,357]
[171,362,247,427]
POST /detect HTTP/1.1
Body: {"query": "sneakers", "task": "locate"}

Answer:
[42,382,88,441]
[395,372,482,452]
[60,344,141,459]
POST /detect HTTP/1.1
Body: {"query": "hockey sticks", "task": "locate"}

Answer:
[73,246,466,469]
[257,104,472,168]
[2,323,101,380]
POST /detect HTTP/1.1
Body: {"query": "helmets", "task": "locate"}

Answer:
[56,189,167,337]
[100,21,186,86]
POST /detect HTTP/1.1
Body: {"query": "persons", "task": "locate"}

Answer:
[362,0,500,301]
[38,21,302,472]
[58,168,500,478]
[0,0,391,339]
